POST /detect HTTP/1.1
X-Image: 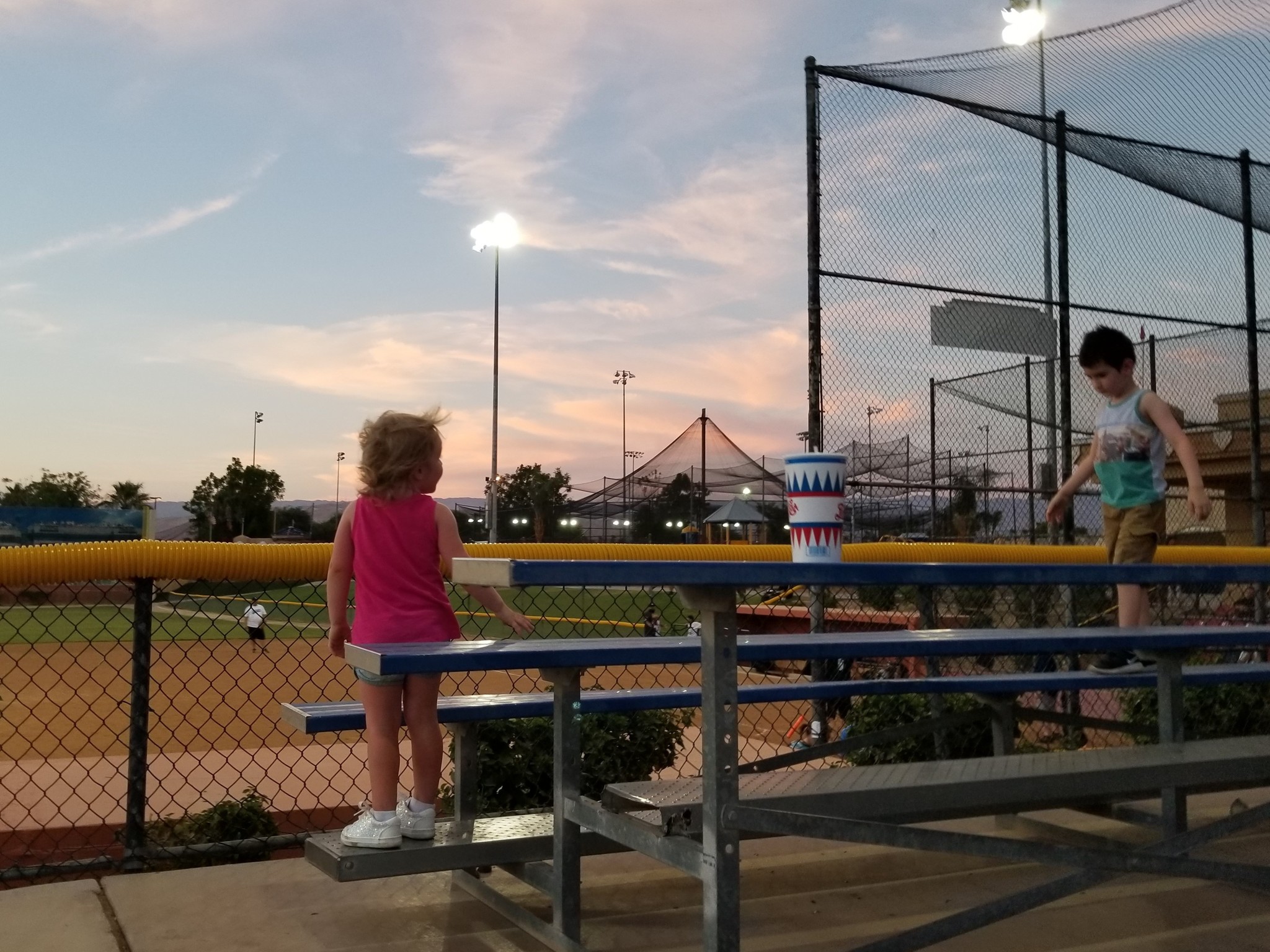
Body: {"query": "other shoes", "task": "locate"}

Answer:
[264,648,270,653]
[252,648,257,652]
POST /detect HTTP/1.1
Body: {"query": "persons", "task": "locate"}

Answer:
[327,406,533,847]
[1234,587,1255,620]
[243,598,269,653]
[685,615,701,636]
[838,725,857,757]
[644,604,666,636]
[1047,325,1211,672]
[787,721,832,749]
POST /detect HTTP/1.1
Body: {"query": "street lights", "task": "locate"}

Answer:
[624,450,644,529]
[470,211,523,542]
[978,424,991,534]
[866,405,884,536]
[796,431,809,453]
[612,370,637,543]
[252,411,264,466]
[336,452,345,531]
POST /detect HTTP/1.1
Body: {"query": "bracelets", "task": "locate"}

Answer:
[1188,483,1204,490]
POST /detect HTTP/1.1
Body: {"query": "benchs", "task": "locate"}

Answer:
[279,559,1270,952]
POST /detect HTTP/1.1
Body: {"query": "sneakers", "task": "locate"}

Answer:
[1087,651,1162,674]
[395,797,439,840]
[340,801,404,851]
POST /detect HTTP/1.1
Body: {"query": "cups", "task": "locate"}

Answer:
[784,451,848,562]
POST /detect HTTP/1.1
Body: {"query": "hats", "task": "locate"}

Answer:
[251,596,258,602]
